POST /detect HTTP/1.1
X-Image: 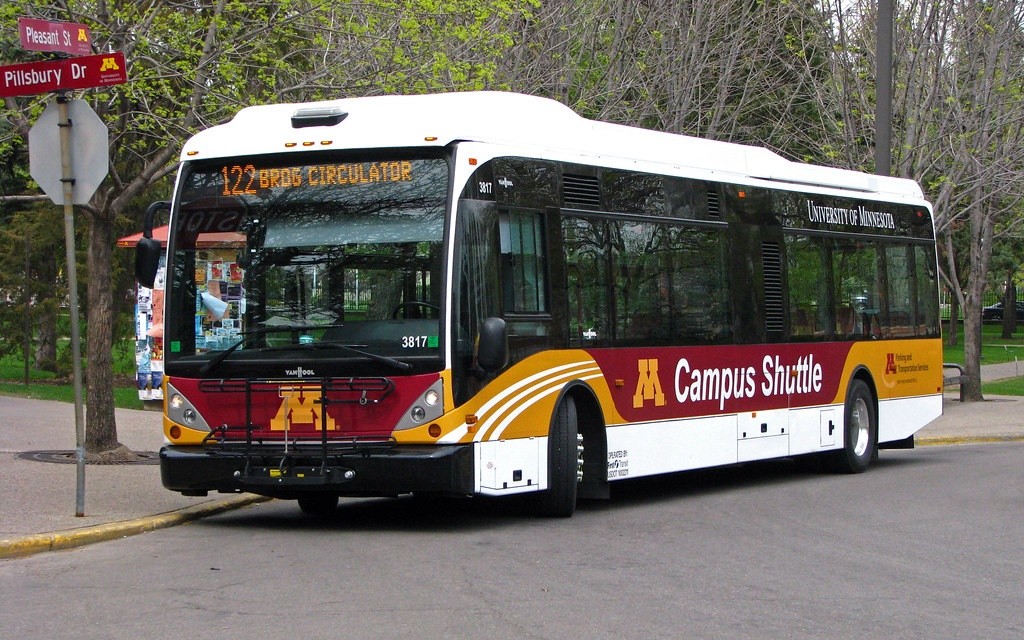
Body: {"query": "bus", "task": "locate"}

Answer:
[132,91,944,520]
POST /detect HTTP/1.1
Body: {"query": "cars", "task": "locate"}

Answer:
[851,287,870,310]
[982,301,1024,319]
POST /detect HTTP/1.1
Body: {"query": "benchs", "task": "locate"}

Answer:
[942,362,969,415]
